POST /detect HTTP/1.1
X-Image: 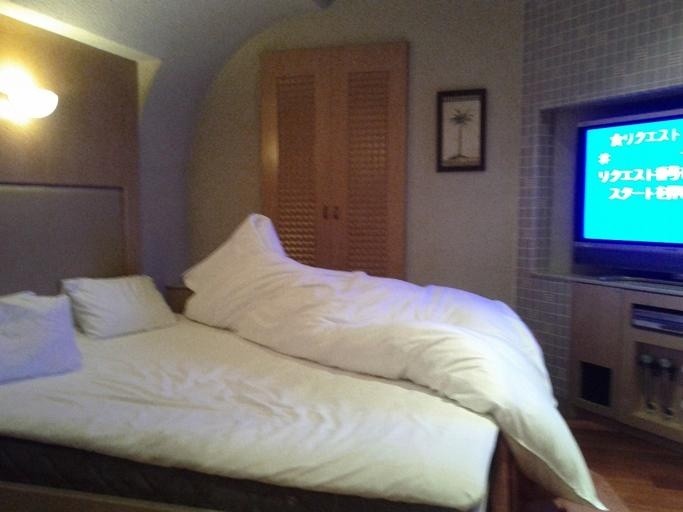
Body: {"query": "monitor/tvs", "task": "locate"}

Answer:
[570,108,683,287]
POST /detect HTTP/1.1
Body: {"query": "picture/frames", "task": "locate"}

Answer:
[435,88,486,172]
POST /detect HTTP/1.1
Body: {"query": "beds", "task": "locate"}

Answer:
[1,181,612,512]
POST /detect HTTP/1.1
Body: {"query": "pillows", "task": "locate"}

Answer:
[2,289,38,297]
[64,274,178,342]
[0,291,87,387]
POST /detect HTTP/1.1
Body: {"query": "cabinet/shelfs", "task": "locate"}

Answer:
[568,278,682,442]
[262,39,409,280]
